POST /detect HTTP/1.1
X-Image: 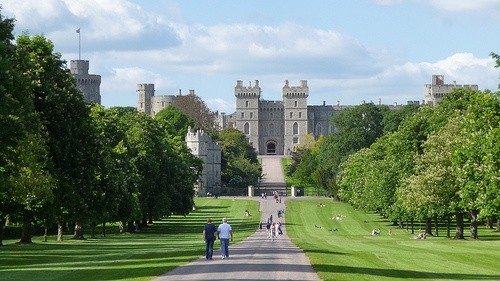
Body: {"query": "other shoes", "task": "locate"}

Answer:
[222,256,228,259]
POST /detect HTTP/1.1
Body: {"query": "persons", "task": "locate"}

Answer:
[217,216,233,260]
[266,215,283,238]
[372,228,380,235]
[417,233,426,239]
[207,191,218,199]
[202,218,217,260]
[278,210,282,218]
[273,190,282,203]
[260,221,263,231]
[262,192,267,198]
